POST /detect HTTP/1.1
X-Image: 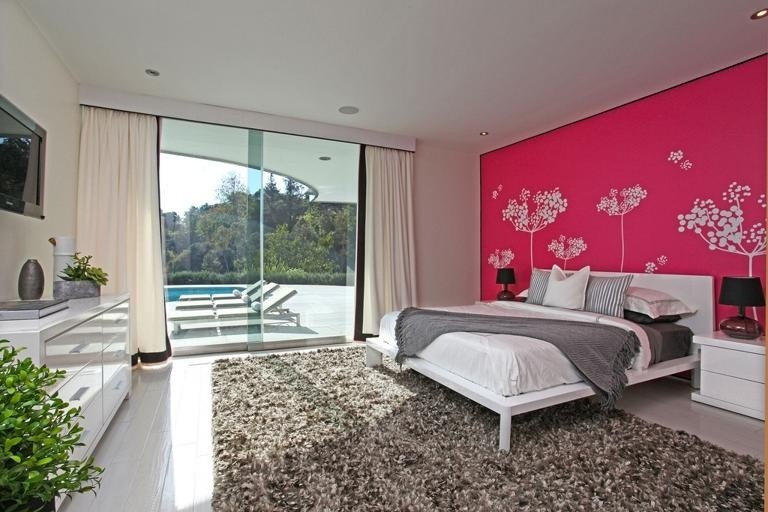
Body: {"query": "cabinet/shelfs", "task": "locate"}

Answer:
[0,294,132,511]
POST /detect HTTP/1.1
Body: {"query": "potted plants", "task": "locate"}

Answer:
[1,339,105,511]
[51,254,108,302]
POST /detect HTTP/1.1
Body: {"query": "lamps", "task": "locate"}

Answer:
[495,268,515,300]
[718,275,766,339]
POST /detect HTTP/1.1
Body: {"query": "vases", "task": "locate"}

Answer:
[18,259,44,300]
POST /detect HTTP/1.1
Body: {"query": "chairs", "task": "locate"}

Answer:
[168,279,300,339]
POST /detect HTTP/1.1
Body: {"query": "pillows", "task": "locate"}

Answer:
[525,263,698,325]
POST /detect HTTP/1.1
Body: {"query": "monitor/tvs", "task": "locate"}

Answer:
[0,94,47,220]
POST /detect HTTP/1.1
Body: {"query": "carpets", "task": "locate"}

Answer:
[212,347,764,511]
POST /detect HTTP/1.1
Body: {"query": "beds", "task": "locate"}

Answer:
[365,267,715,452]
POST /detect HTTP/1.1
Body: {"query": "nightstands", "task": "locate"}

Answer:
[690,331,765,420]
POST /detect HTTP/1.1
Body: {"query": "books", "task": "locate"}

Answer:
[1,299,68,321]
